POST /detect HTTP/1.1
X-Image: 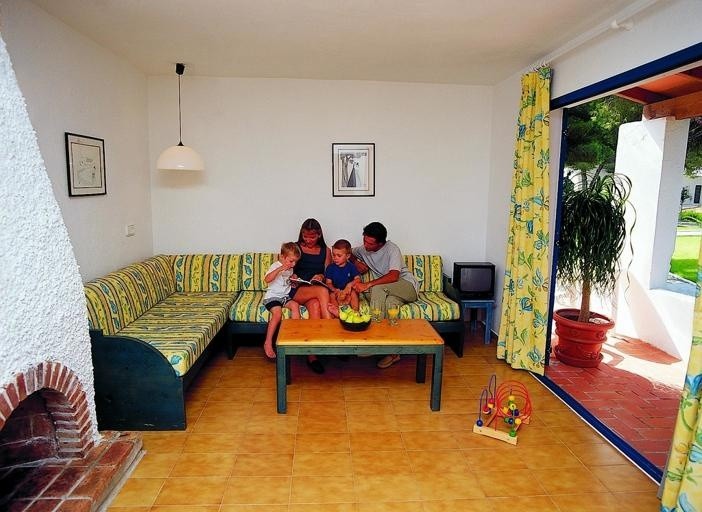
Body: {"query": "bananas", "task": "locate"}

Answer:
[341,305,352,313]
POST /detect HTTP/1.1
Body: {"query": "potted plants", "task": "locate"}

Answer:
[553,168,638,369]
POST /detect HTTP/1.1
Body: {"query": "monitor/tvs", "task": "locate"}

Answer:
[453,262,495,299]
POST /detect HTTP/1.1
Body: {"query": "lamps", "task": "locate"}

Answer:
[155,62,205,172]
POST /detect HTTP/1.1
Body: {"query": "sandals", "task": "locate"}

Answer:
[263,342,277,360]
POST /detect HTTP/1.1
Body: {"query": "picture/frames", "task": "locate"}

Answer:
[63,131,107,197]
[330,142,376,197]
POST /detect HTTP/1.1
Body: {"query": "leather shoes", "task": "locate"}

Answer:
[307,358,324,374]
[377,355,400,368]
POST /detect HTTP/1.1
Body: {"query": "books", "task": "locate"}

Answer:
[290,278,328,287]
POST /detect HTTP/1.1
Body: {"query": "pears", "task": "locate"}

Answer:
[340,313,371,323]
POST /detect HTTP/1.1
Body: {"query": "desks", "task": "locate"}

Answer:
[274,317,446,415]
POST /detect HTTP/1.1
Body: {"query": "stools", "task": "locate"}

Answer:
[460,300,497,345]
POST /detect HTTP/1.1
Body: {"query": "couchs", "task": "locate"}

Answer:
[224,252,466,359]
[82,254,242,433]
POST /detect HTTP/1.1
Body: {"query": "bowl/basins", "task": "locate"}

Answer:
[339,317,371,331]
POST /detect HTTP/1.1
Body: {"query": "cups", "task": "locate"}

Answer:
[386,304,400,327]
[370,301,385,325]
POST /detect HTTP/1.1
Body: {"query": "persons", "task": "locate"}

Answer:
[262,242,301,359]
[325,239,362,318]
[348,222,420,367]
[289,219,333,374]
